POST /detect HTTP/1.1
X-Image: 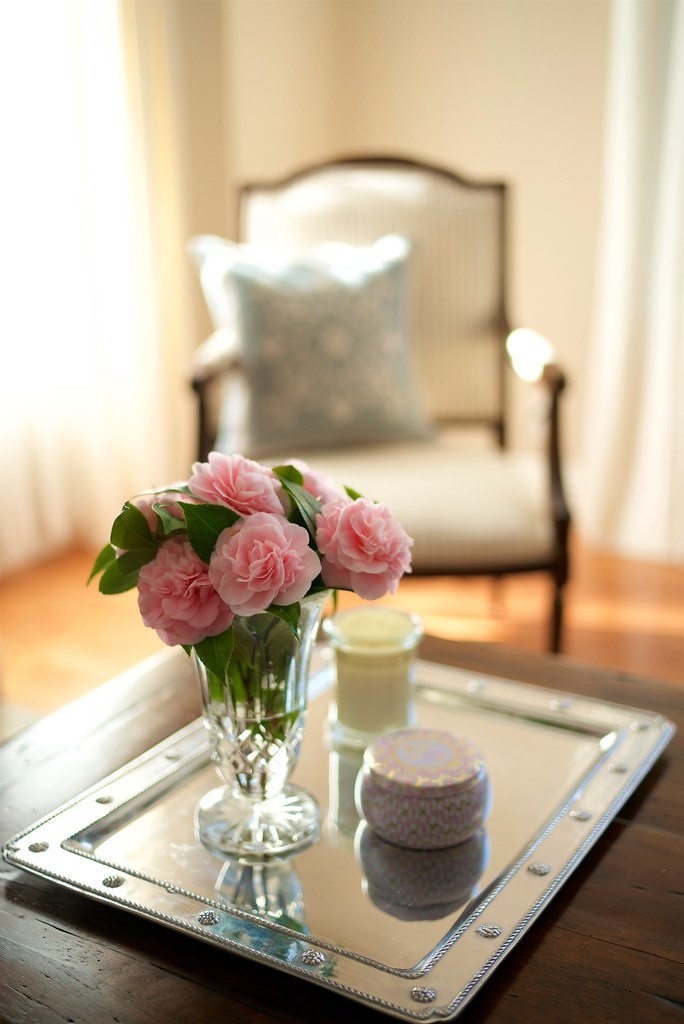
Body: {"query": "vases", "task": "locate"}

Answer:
[192,592,330,854]
[218,859,311,936]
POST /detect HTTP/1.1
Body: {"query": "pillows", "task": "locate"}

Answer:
[191,232,427,459]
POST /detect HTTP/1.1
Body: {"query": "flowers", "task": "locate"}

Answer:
[84,448,413,803]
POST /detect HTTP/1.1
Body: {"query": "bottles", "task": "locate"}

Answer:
[354,729,491,849]
[353,824,489,921]
[325,605,423,752]
[324,747,365,833]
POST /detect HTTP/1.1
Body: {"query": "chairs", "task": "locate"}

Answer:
[191,152,570,653]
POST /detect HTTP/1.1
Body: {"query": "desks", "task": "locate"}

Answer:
[0,635,684,1024]
